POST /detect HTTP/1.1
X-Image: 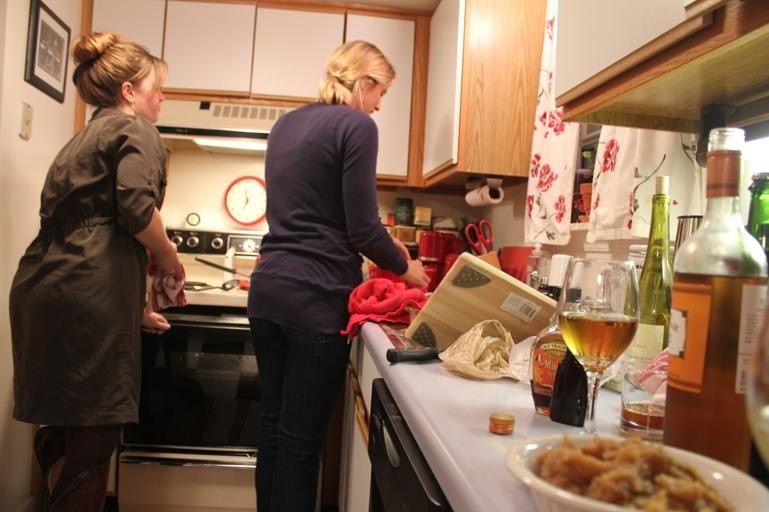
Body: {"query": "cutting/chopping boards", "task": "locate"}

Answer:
[403,252,556,351]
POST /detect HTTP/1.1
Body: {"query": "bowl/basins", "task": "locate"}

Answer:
[506,434,768,512]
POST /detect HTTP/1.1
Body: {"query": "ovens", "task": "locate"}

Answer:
[113,315,261,466]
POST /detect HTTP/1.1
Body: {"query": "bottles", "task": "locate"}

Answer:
[641,174,672,351]
[526,243,546,291]
[662,127,769,472]
[528,308,586,428]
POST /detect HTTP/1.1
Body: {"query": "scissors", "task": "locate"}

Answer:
[465,220,493,254]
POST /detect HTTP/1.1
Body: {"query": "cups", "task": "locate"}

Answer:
[620,360,666,438]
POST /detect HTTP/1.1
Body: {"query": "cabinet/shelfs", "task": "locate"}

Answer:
[554,0,769,135]
[160,2,344,109]
[415,1,545,194]
[85,1,162,62]
[344,0,417,196]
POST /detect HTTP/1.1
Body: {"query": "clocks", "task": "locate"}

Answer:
[223,176,266,226]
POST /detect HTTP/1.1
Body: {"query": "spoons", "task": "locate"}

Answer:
[192,278,240,293]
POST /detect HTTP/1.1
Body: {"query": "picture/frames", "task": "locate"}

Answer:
[24,0,72,103]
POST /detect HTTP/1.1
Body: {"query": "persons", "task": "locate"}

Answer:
[9,33,186,511]
[247,41,431,512]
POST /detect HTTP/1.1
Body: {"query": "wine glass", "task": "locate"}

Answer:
[558,257,639,437]
